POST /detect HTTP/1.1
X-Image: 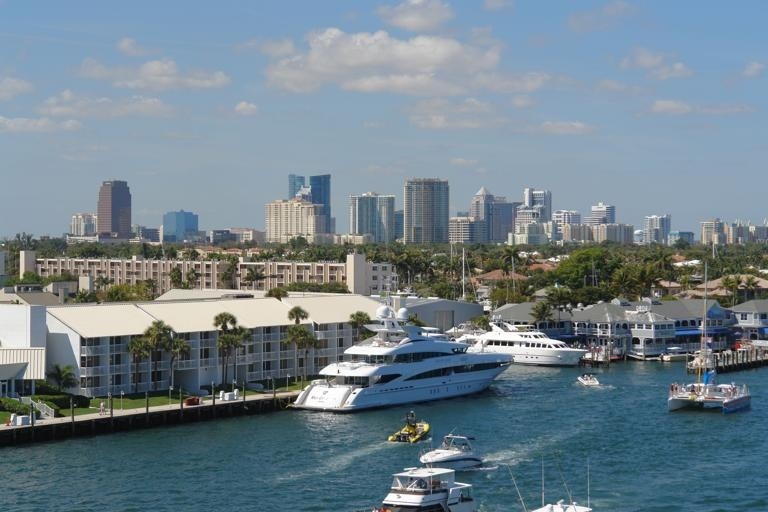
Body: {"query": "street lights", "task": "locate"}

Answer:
[231,379,238,392]
[169,386,175,408]
[267,375,272,393]
[285,371,291,392]
[107,391,111,415]
[120,389,125,413]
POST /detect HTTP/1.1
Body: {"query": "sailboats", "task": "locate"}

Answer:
[665,259,754,415]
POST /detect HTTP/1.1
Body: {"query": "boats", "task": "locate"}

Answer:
[659,345,689,363]
[464,313,590,371]
[576,372,600,386]
[369,411,593,512]
[286,304,519,414]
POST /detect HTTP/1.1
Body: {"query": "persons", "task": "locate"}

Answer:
[667,380,739,401]
[97,400,109,416]
